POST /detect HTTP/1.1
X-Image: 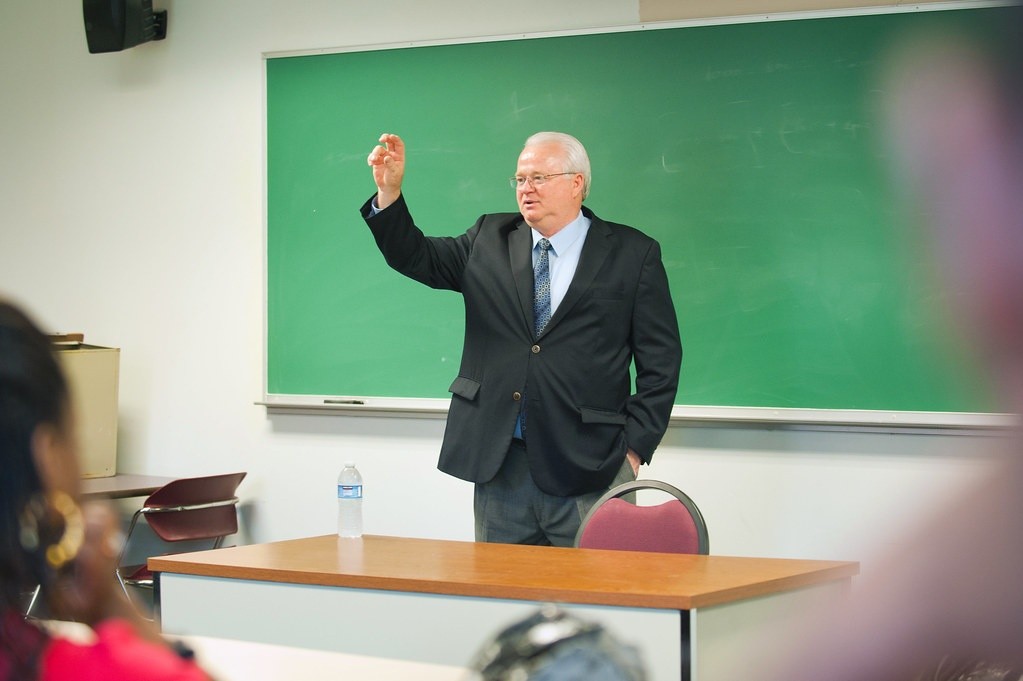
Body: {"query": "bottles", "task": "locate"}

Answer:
[336,461,363,538]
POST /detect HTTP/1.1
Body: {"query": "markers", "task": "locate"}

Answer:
[324,399,364,404]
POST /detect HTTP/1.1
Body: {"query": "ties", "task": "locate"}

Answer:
[520,238,553,441]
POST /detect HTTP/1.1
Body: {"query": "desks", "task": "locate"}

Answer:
[139,535,862,681]
[21,477,195,617]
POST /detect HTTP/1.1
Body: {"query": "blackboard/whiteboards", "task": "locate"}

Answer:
[262,1,1023,426]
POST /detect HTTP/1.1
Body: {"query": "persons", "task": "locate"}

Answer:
[0,303,216,681]
[358,132,682,548]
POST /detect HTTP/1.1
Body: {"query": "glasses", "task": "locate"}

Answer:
[508,172,578,190]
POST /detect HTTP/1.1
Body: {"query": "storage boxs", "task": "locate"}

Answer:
[49,343,121,477]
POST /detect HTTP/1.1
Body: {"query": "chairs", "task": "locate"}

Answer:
[571,478,710,558]
[116,470,245,621]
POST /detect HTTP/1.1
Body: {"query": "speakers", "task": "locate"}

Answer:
[82,0,155,54]
